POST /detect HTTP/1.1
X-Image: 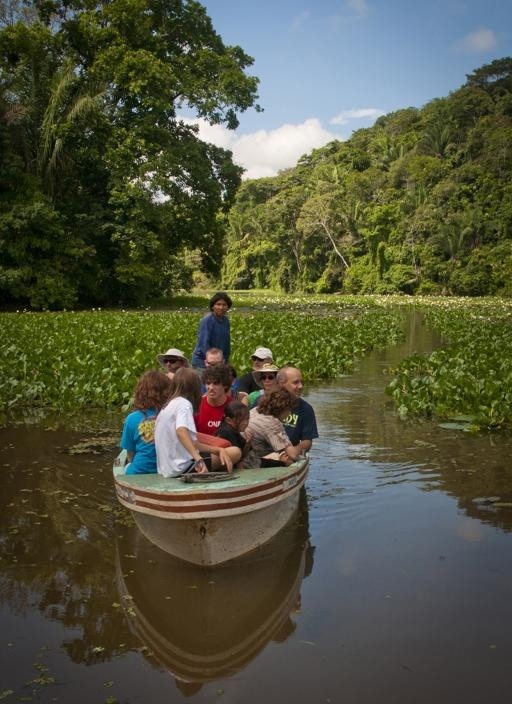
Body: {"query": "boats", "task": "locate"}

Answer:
[111,448,310,569]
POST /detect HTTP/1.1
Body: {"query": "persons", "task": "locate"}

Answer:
[120,292,319,475]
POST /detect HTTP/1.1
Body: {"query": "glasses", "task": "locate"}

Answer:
[252,356,264,362]
[163,358,182,364]
[260,374,275,380]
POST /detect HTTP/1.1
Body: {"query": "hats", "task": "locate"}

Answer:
[252,363,281,389]
[252,348,272,361]
[156,348,189,369]
